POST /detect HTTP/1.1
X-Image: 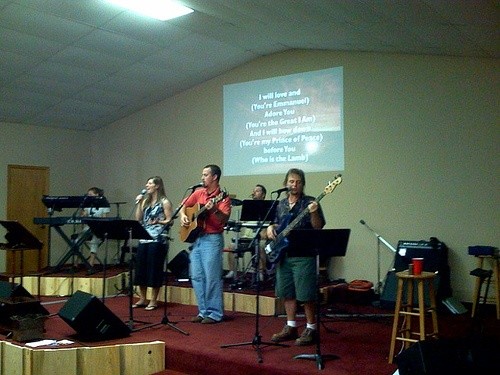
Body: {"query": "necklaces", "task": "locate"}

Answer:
[288,199,298,204]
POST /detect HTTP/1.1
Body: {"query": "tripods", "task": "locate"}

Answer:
[86,188,197,336]
[219,193,351,369]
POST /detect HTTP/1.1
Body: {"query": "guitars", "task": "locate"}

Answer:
[180,187,229,244]
[262,172,343,264]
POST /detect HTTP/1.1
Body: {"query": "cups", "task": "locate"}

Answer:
[408,258,423,275]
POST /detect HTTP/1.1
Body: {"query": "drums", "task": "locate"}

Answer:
[237,237,256,251]
[223,220,243,232]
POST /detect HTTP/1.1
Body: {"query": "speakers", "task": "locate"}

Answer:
[381,270,438,307]
[397,336,489,375]
[0,281,49,324]
[57,291,131,340]
[393,240,451,310]
[167,250,191,281]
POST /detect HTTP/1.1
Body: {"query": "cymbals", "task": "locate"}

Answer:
[230,197,242,207]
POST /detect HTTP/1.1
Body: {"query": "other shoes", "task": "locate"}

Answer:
[257,272,264,282]
[192,316,218,323]
[225,270,234,278]
[295,328,319,345]
[272,324,298,342]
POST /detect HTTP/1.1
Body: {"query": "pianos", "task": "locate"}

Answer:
[32,193,122,276]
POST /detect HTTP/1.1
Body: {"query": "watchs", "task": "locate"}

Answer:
[214,209,219,215]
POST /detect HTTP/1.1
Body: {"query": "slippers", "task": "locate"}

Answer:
[144,303,159,311]
[132,303,147,307]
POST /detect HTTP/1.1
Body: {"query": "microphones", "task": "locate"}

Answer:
[135,189,146,204]
[272,188,288,193]
[192,185,203,189]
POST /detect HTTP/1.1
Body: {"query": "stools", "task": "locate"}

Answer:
[471,255,500,319]
[388,272,440,363]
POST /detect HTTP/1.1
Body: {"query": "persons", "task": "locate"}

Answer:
[132,176,174,310]
[223,185,269,281]
[267,169,325,345]
[70,187,111,274]
[178,165,231,323]
